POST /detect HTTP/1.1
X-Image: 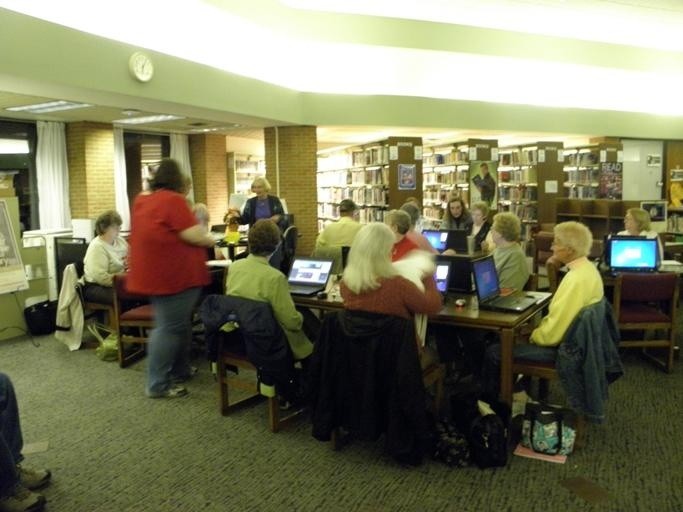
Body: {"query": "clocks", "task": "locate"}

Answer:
[128,51,153,83]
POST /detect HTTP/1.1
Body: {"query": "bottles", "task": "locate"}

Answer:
[481,240,488,256]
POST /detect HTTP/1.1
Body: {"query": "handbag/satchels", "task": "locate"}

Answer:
[430,404,510,469]
[520,399,579,457]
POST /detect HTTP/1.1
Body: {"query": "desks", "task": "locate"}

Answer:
[285,277,553,469]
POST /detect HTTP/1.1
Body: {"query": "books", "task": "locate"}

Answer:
[562,152,601,199]
[494,148,537,218]
[313,144,385,231]
[655,258,682,272]
[422,145,471,229]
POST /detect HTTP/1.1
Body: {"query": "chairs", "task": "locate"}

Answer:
[529,234,562,295]
[204,211,299,289]
[511,298,629,431]
[605,271,681,375]
[52,236,113,358]
[313,308,448,466]
[112,273,160,368]
[202,291,313,434]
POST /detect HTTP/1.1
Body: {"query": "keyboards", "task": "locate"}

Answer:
[612,271,658,276]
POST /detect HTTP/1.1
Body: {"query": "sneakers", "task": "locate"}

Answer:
[15,459,52,492]
[145,382,189,398]
[168,370,185,384]
[0,482,47,512]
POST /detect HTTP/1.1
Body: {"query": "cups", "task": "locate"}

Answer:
[465,236,475,254]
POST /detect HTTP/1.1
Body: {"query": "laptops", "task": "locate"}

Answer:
[472,253,539,314]
[342,246,351,269]
[286,255,335,298]
[434,255,477,294]
[210,224,227,233]
[438,228,468,254]
[610,239,658,269]
[433,261,449,304]
[421,229,449,254]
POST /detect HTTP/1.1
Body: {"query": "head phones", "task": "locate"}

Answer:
[224,210,241,225]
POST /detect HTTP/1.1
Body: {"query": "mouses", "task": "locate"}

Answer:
[317,291,328,299]
[455,299,466,308]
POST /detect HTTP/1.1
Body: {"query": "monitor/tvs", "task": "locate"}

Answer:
[604,235,646,265]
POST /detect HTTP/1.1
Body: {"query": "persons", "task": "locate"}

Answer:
[223,217,325,373]
[227,176,286,239]
[186,203,216,261]
[307,196,532,390]
[473,219,607,417]
[0,373,52,512]
[124,158,226,399]
[79,209,152,352]
[611,207,664,268]
[472,163,495,206]
